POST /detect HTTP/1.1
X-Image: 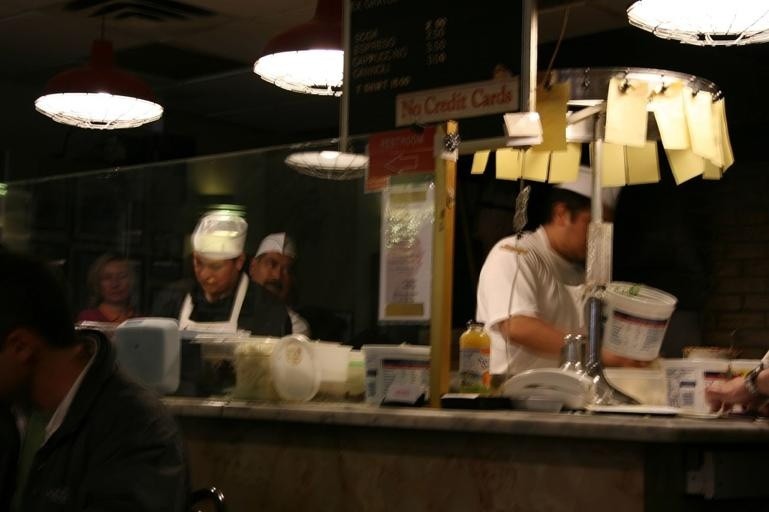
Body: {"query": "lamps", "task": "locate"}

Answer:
[252,0,346,98]
[626,1,769,47]
[32,0,165,131]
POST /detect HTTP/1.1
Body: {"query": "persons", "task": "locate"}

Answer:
[245,231,311,341]
[473,160,650,386]
[704,345,769,422]
[0,239,192,512]
[76,251,141,327]
[160,208,291,398]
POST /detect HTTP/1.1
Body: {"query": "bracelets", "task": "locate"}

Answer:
[744,363,764,398]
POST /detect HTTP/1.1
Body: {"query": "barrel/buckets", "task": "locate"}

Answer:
[663,357,729,414]
[360,344,431,407]
[601,283,677,368]
[729,360,769,413]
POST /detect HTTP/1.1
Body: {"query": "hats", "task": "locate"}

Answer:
[553,166,623,209]
[192,212,248,260]
[255,233,298,259]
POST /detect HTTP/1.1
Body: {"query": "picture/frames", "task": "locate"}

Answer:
[376,180,435,328]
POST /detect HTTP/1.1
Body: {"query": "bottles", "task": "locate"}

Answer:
[459,320,492,393]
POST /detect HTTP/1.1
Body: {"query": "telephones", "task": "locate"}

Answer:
[502,368,592,411]
